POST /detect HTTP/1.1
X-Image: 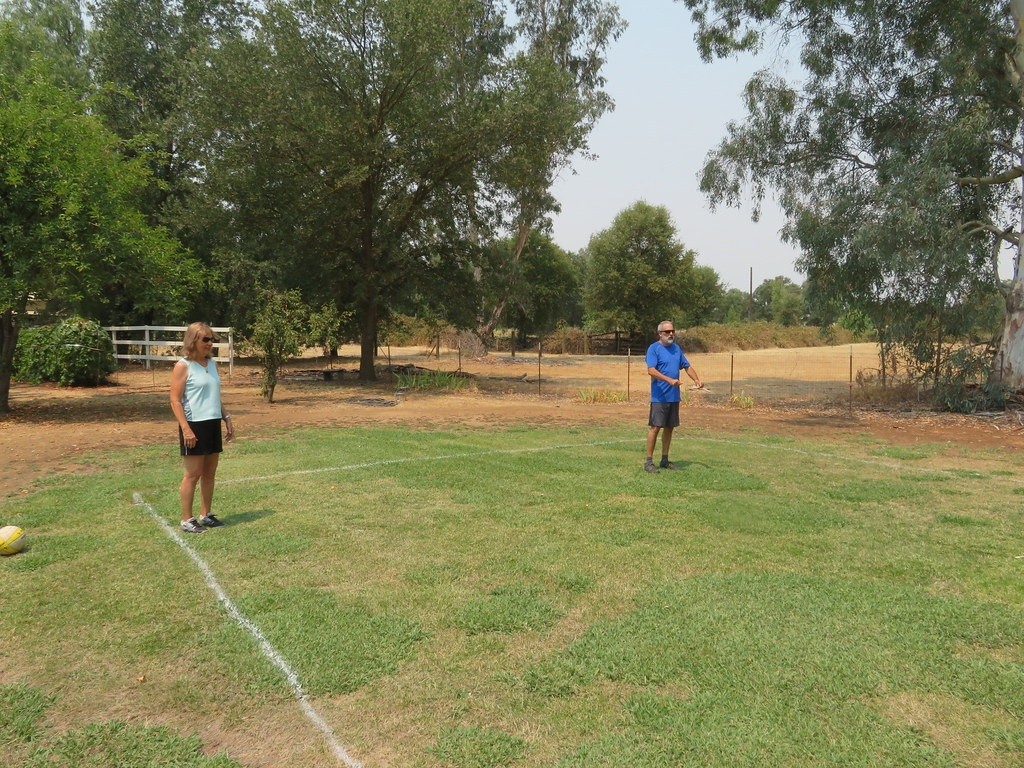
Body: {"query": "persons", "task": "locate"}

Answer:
[170,322,234,532]
[644,321,704,472]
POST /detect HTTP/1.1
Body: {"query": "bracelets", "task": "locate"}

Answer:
[224,414,231,421]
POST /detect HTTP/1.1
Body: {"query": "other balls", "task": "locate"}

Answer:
[0,526,26,555]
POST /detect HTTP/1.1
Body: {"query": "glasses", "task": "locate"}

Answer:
[198,337,215,342]
[660,330,675,333]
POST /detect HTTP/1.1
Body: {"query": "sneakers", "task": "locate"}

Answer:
[198,512,225,530]
[181,517,208,534]
[644,462,660,473]
[660,462,677,470]
